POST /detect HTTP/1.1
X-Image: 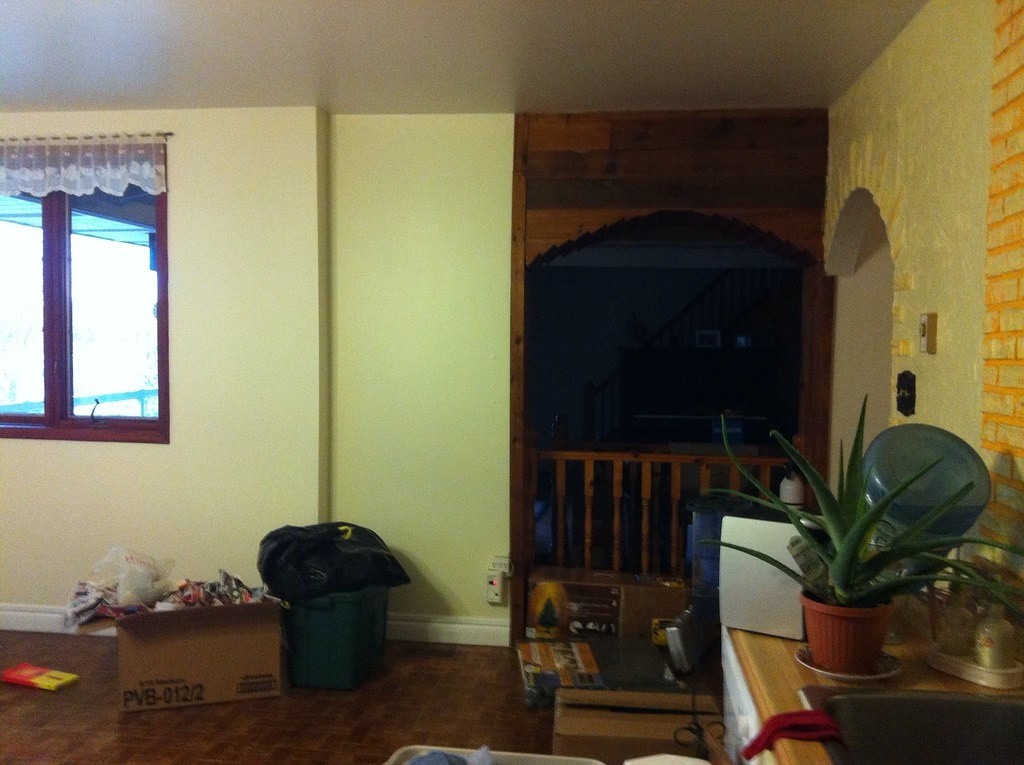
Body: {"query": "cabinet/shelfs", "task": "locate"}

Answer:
[722,588,1024,765]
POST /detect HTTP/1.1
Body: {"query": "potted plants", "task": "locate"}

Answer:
[701,392,1023,677]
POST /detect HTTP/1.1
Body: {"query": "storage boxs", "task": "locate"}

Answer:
[382,745,605,765]
[282,585,387,689]
[552,688,723,764]
[78,546,292,714]
[527,567,688,646]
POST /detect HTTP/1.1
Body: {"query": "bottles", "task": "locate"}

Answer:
[937,595,974,655]
[975,596,1016,670]
[858,423,992,592]
